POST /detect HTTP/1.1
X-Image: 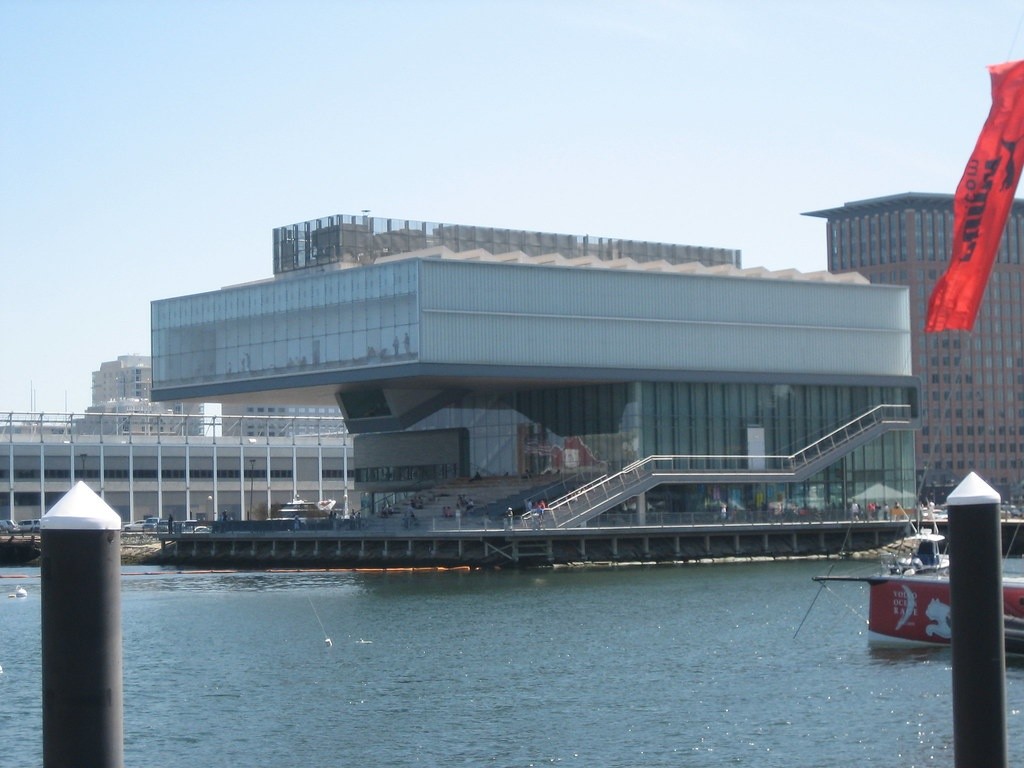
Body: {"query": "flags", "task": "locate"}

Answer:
[922,58,1024,333]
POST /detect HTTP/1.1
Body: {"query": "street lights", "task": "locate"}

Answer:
[249,459,256,521]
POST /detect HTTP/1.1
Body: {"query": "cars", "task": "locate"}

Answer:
[123,518,210,533]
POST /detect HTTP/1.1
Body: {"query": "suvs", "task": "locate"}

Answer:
[18,519,41,533]
[0,520,21,533]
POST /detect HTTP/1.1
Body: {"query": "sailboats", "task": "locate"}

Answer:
[812,14,1024,656]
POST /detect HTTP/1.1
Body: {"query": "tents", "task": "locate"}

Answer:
[841,482,915,500]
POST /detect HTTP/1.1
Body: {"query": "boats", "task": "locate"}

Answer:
[878,502,951,577]
[264,497,337,522]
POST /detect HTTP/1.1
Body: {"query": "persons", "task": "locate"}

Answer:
[168,514,173,533]
[367,347,387,357]
[472,473,482,480]
[528,498,545,530]
[443,493,477,518]
[329,509,362,530]
[719,502,902,522]
[312,341,320,364]
[286,356,306,368]
[220,509,230,531]
[381,505,394,516]
[507,507,514,530]
[295,514,300,530]
[392,335,400,356]
[410,496,423,509]
[404,332,410,353]
